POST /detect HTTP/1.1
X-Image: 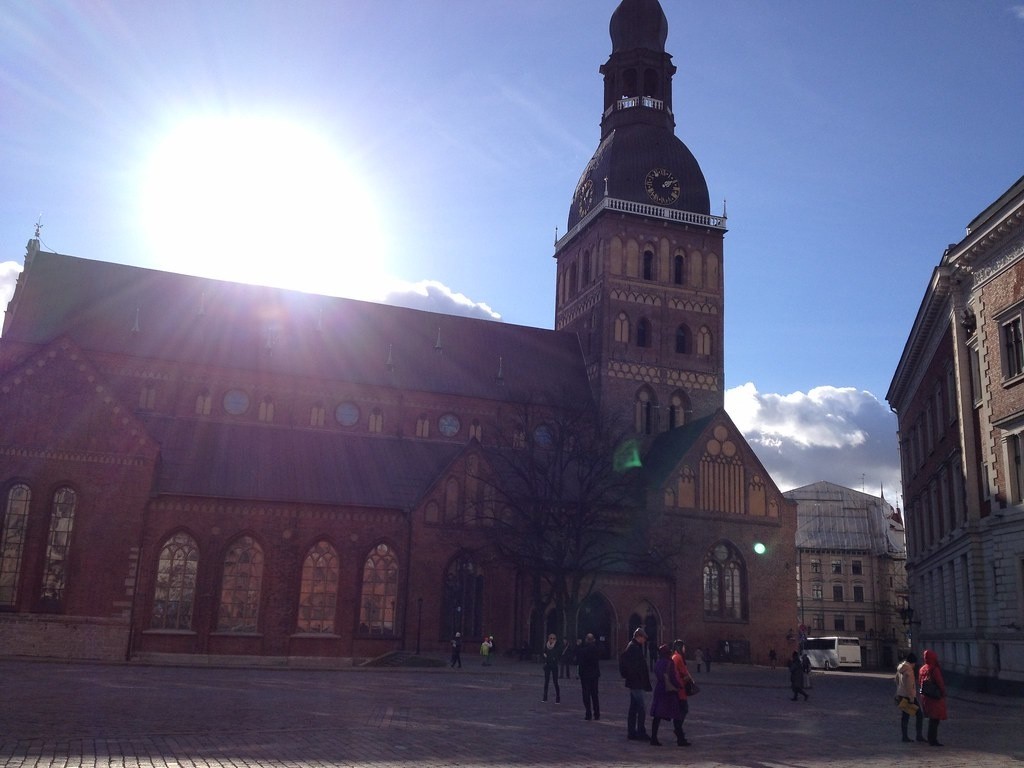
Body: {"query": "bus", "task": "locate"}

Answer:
[799,636,862,671]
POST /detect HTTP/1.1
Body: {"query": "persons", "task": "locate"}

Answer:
[896,653,927,742]
[770,649,811,702]
[918,650,948,745]
[451,632,462,669]
[541,634,600,721]
[480,635,495,666]
[621,627,711,746]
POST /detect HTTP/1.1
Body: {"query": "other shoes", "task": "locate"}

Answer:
[902,737,914,741]
[930,741,944,746]
[804,694,810,701]
[584,715,600,720]
[541,700,548,703]
[650,738,663,746]
[627,733,651,741]
[916,736,927,742]
[790,696,798,701]
[555,701,560,704]
[677,739,692,746]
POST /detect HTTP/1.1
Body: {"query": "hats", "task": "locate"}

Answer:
[659,644,670,652]
[585,633,595,643]
[455,632,460,637]
[635,627,648,639]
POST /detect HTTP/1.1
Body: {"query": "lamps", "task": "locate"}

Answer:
[906,604,922,626]
[900,605,913,627]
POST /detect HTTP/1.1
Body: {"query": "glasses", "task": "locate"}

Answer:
[549,637,556,640]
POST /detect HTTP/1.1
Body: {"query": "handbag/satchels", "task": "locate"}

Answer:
[919,663,944,700]
[897,698,920,717]
[663,660,681,693]
[685,679,700,696]
[641,668,653,692]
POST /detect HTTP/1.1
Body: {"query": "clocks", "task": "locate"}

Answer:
[644,167,681,206]
[576,179,595,216]
